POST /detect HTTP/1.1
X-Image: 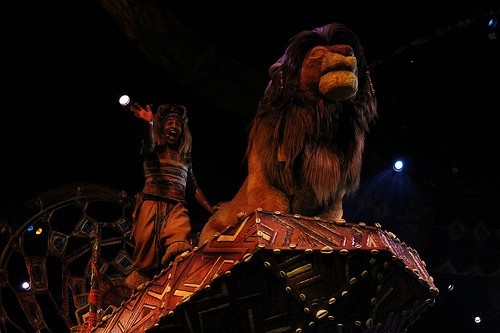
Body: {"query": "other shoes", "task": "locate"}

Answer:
[174,246,199,262]
[125,271,149,290]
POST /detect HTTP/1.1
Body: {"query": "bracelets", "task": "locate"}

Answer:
[147,119,154,126]
[202,200,208,206]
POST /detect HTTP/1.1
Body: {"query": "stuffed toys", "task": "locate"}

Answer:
[198,20,379,250]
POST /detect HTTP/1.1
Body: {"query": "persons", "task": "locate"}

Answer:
[129,101,228,288]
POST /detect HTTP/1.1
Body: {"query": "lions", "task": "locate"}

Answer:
[199,22,379,246]
